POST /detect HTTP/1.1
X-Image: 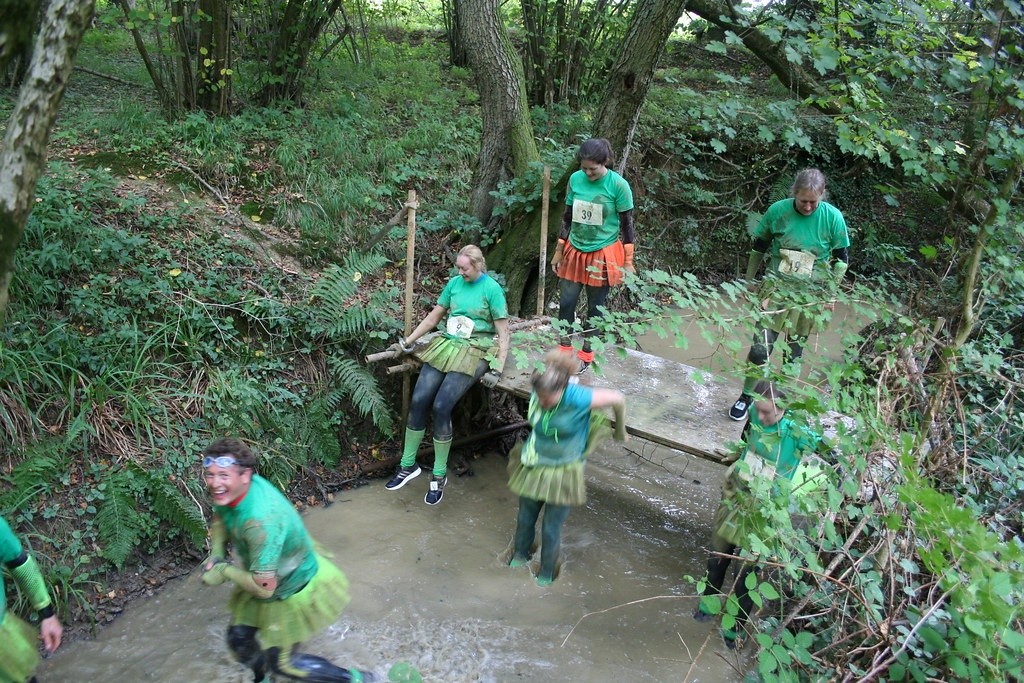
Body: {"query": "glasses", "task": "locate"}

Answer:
[202,457,244,468]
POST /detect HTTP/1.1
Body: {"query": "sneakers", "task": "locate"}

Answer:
[694,605,715,622]
[424,474,449,505]
[574,350,594,375]
[725,630,748,650]
[385,461,421,490]
[729,392,755,422]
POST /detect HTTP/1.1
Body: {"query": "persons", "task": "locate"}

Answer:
[198,437,376,683]
[692,377,853,650]
[727,167,851,421]
[507,349,631,585]
[549,137,634,375]
[0,515,63,683]
[384,244,510,506]
